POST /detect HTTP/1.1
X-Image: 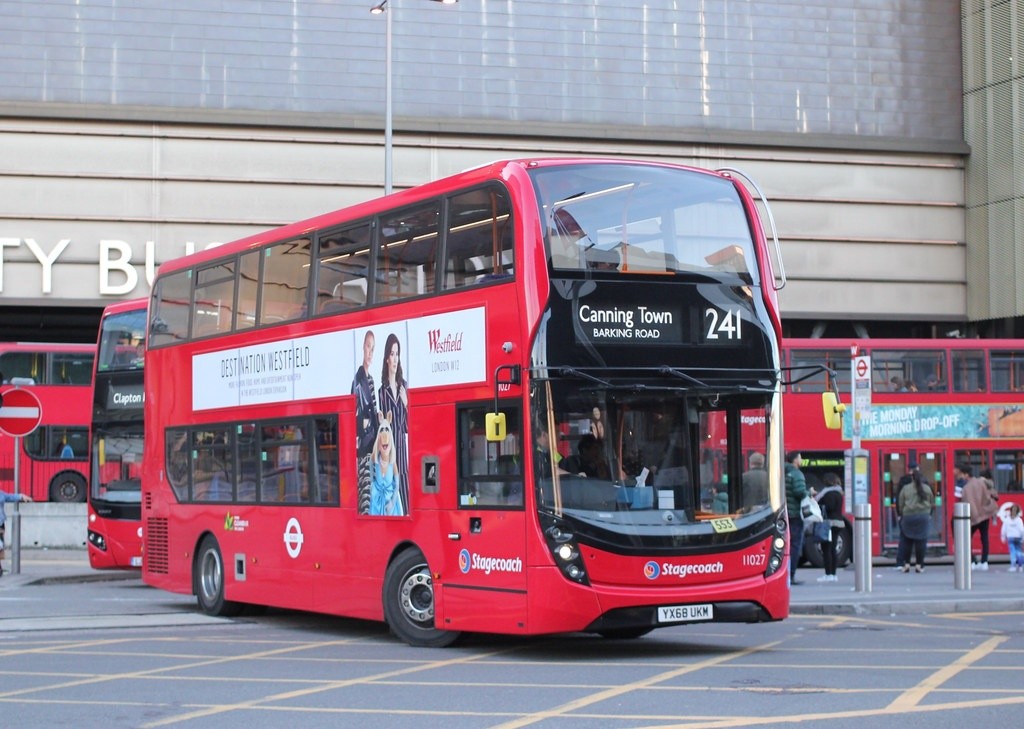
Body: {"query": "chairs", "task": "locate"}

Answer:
[176,462,338,502]
[165,296,361,342]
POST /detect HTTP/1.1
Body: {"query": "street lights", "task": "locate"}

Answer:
[370,1,460,197]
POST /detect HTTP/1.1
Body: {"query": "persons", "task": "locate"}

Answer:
[951,464,979,569]
[507,421,588,504]
[813,474,845,581]
[351,330,408,516]
[784,451,817,584]
[0,490,31,576]
[742,453,768,507]
[712,483,728,513]
[610,398,683,506]
[566,434,611,480]
[130,342,145,369]
[899,471,933,573]
[1001,505,1024,571]
[894,462,929,569]
[961,466,998,570]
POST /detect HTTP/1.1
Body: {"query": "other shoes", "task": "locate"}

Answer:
[1018,566,1023,572]
[898,563,925,572]
[1008,567,1017,571]
[791,579,800,585]
[816,574,837,581]
[972,561,988,570]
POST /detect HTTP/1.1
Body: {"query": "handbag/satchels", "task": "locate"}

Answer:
[812,505,832,543]
[799,489,824,523]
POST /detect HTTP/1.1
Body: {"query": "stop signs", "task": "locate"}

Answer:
[1,388,42,437]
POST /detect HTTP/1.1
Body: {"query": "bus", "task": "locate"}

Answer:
[141,157,851,649]
[699,337,1024,568]
[86,296,153,569]
[0,341,135,501]
